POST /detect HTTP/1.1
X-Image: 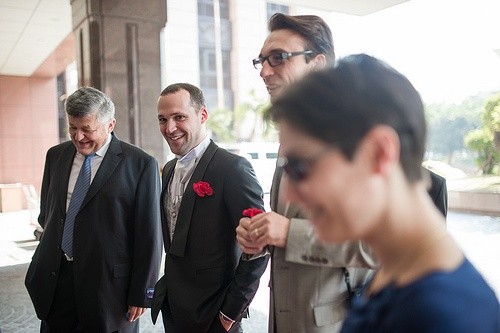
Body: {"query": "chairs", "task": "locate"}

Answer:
[22,184,41,227]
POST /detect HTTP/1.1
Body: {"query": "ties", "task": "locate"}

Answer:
[60,154,99,259]
[173,157,200,202]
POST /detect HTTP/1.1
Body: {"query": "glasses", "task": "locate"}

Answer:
[282,143,345,181]
[254,49,313,71]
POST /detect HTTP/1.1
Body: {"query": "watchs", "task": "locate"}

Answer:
[219,312,235,323]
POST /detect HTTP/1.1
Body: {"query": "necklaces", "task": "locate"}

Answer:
[354,232,428,297]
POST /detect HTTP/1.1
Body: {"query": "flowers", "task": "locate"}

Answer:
[242,208,264,218]
[192,180,214,197]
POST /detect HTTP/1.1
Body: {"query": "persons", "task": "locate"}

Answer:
[150,83,275,333]
[263,54,500,333]
[236,13,377,333]
[24,86,162,333]
[419,164,448,219]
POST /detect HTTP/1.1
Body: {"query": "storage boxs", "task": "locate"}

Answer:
[0,183,23,213]
